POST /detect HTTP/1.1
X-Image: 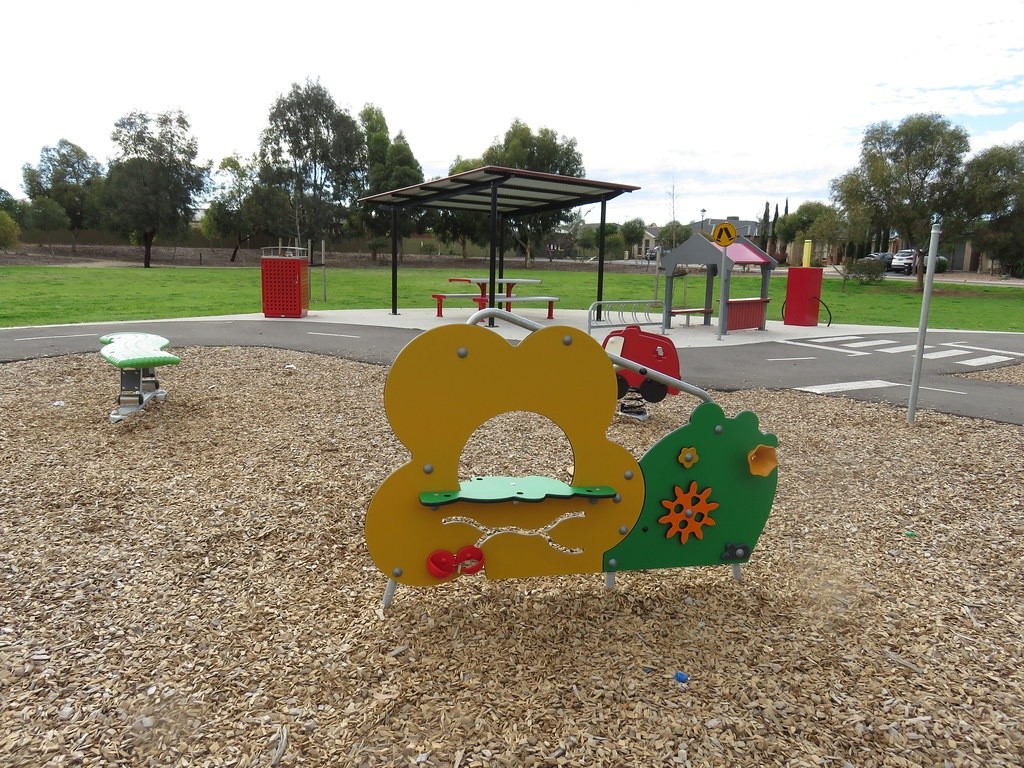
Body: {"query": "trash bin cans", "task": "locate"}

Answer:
[260,246,310,319]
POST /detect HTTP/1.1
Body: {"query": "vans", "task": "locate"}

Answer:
[645,249,656,260]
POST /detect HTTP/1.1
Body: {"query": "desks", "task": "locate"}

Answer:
[448,278,542,322]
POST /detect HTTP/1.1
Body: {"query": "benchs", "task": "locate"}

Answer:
[716,298,772,330]
[432,293,560,322]
[99,332,181,423]
[671,308,711,327]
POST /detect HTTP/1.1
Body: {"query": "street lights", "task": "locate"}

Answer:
[700,208,706,231]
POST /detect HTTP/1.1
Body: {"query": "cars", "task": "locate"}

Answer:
[856,252,896,273]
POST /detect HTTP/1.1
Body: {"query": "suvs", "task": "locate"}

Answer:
[891,249,948,274]
